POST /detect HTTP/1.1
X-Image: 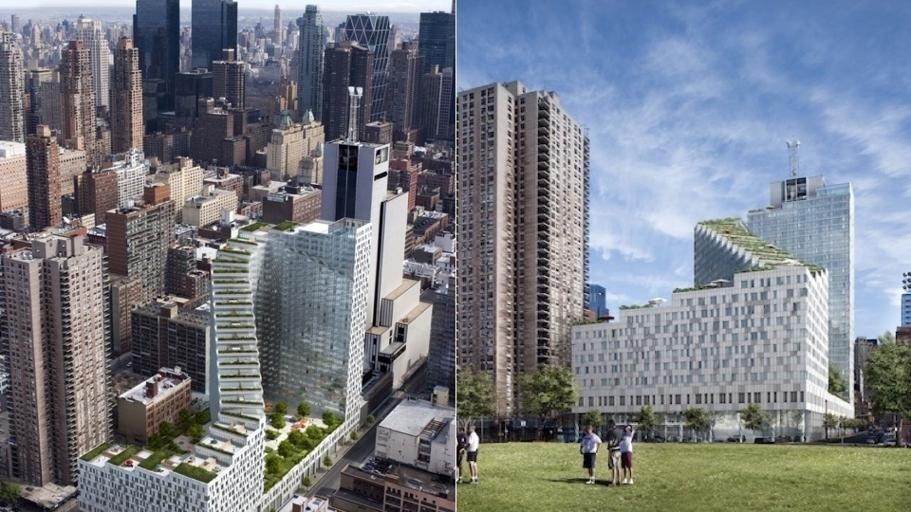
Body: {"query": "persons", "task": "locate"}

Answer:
[580,425,602,484]
[607,419,623,487]
[467,424,480,483]
[622,425,636,485]
[457,426,467,483]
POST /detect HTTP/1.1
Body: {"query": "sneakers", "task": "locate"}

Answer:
[586,480,595,485]
[470,478,478,484]
[612,478,633,486]
